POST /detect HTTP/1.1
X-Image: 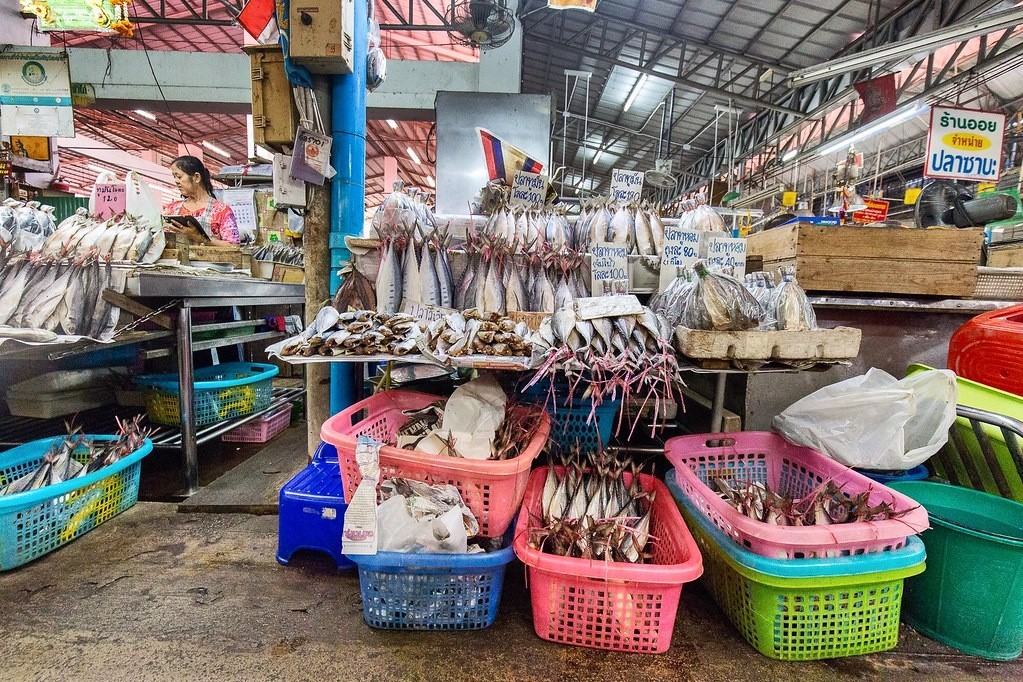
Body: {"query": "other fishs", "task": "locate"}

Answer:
[0,206,167,343]
[278,185,821,406]
[376,391,933,653]
[241,233,306,268]
[0,408,151,555]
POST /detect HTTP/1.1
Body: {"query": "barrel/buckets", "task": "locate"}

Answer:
[876,478,1022,664]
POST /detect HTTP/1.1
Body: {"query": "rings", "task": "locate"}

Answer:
[180,225,183,229]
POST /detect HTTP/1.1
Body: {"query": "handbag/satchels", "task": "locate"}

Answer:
[89,170,161,223]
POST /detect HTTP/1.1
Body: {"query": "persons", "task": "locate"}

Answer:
[163,156,239,247]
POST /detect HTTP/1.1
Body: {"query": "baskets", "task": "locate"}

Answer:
[321,387,549,537]
[664,430,927,661]
[346,517,516,631]
[514,464,705,654]
[220,402,292,443]
[516,371,622,457]
[128,361,279,426]
[973,265,1023,299]
[0,435,153,571]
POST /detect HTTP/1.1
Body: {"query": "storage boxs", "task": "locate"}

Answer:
[0,219,1022,663]
